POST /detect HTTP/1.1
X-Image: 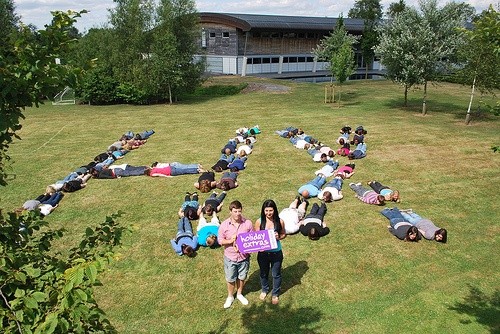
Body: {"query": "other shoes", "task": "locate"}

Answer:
[236,294,248,306]
[224,296,235,309]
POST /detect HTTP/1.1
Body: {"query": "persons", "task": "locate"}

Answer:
[398,209,447,243]
[254,200,286,305]
[367,180,400,203]
[276,124,367,201]
[299,200,330,240]
[217,201,254,309]
[278,196,309,235]
[380,207,421,241]
[14,129,207,220]
[170,124,261,259]
[349,183,385,205]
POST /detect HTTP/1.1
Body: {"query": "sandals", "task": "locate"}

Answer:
[272,296,279,304]
[259,292,267,299]
